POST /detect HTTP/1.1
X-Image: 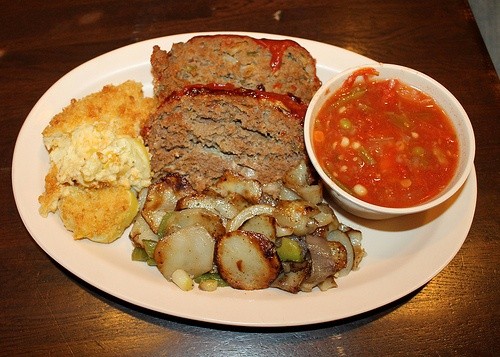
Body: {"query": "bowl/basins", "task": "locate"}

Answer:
[304,62,475,220]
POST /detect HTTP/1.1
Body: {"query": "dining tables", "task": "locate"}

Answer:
[1,0,500,356]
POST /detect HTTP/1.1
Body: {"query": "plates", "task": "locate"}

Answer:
[11,30,477,327]
